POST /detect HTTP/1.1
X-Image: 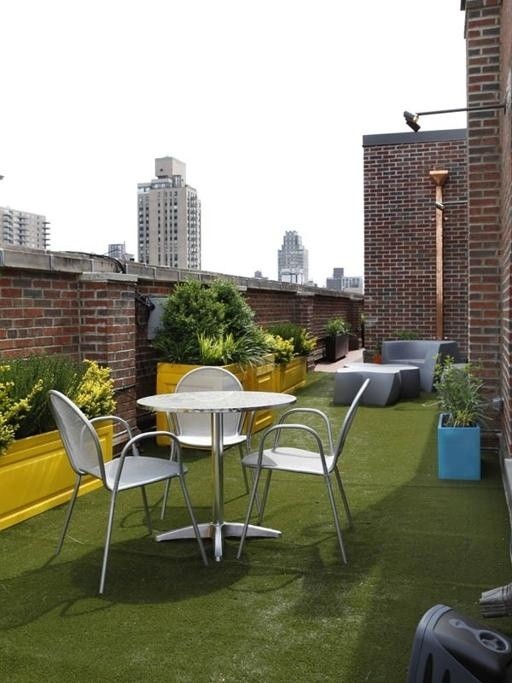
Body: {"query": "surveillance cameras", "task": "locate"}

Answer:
[402,110,420,133]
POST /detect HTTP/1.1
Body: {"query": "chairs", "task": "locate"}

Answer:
[159,365,261,520]
[46,388,208,594]
[235,376,371,564]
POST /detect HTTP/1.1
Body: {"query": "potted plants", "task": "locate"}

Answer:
[324,318,356,362]
[420,350,505,480]
[149,272,300,450]
[267,321,321,395]
[0,350,116,531]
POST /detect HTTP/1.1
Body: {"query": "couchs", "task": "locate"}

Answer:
[381,339,459,392]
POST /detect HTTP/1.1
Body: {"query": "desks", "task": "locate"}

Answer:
[135,390,296,561]
[333,363,419,406]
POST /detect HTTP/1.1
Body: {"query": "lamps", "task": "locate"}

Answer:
[402,100,507,132]
